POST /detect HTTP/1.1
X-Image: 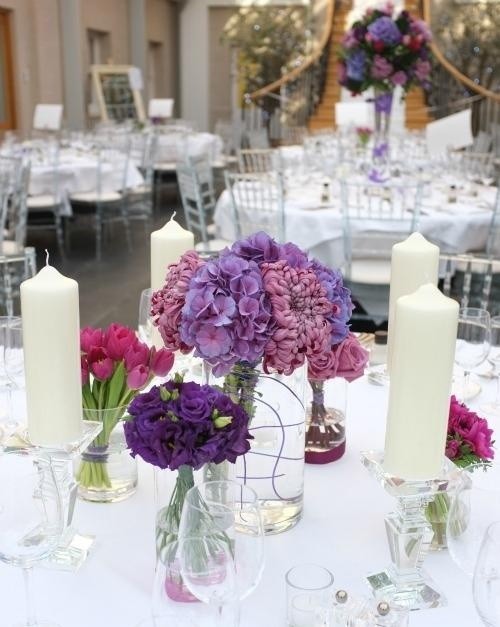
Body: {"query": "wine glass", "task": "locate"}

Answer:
[180,479,266,625]
[300,125,498,216]
[451,306,500,402]
[0,447,66,627]
[0,315,26,439]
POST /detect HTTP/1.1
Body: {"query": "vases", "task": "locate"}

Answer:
[368,74,395,182]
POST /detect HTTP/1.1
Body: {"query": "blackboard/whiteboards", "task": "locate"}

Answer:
[0,257,35,315]
[92,65,144,127]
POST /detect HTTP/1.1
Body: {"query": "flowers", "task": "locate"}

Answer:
[337,0,435,95]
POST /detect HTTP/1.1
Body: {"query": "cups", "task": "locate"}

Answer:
[140,288,196,382]
[445,460,500,626]
[283,562,415,626]
